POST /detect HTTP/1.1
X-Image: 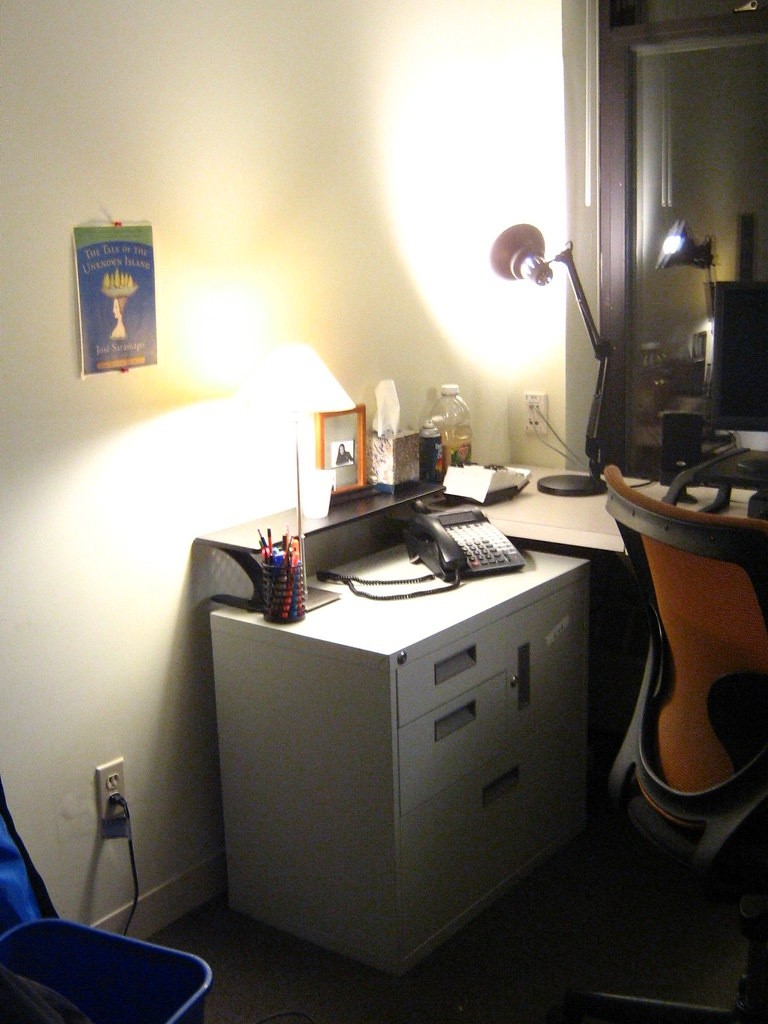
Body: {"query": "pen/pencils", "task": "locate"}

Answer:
[257,528,304,618]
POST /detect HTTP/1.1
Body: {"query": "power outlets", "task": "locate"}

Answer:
[524,392,548,433]
[97,759,127,819]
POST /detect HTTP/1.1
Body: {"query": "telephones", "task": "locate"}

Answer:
[402,507,526,581]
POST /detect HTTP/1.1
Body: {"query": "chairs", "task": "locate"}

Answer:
[546,463,768,1024]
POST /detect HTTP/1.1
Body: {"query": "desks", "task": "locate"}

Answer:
[387,468,757,554]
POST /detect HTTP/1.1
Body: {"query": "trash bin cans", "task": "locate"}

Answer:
[0,919,212,1024]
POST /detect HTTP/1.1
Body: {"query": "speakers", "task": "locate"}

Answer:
[660,412,704,485]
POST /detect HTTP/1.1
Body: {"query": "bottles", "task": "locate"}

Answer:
[419,420,443,483]
[432,416,451,474]
[431,384,471,472]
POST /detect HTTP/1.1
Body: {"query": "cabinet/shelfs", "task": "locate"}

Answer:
[209,540,589,973]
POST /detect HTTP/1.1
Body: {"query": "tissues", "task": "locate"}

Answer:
[366,380,420,494]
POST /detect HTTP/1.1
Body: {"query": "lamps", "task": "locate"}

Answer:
[490,220,615,495]
[656,221,719,421]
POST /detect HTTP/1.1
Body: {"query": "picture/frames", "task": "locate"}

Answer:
[316,403,368,497]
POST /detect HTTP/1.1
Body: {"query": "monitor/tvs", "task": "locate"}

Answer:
[709,278,768,474]
